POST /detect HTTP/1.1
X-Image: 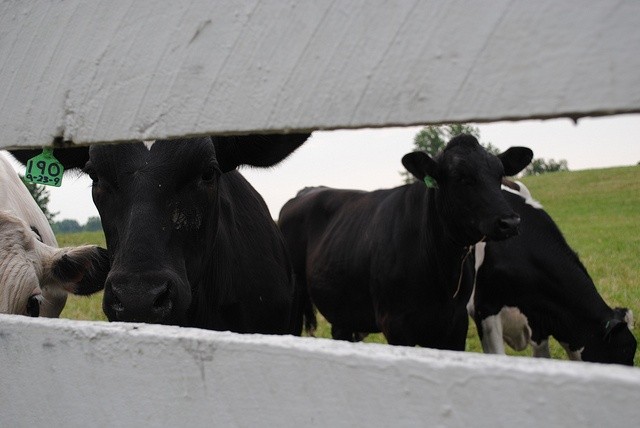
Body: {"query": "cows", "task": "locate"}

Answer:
[276,133,533,351]
[8,132,306,338]
[0,149,111,318]
[466,178,638,366]
